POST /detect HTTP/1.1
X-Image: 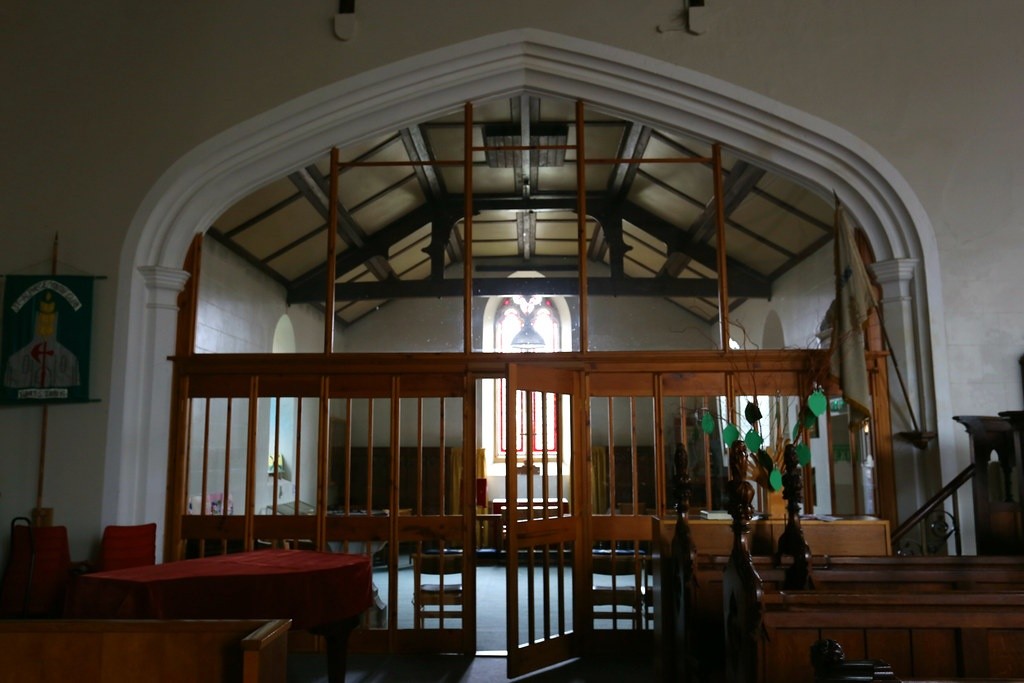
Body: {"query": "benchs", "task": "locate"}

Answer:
[647,508,1024,683]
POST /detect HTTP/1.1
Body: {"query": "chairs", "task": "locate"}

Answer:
[589,548,649,633]
[619,502,646,550]
[412,547,462,632]
[5,523,157,621]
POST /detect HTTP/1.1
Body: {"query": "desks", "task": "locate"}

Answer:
[66,552,373,683]
[329,508,413,564]
[492,498,568,552]
